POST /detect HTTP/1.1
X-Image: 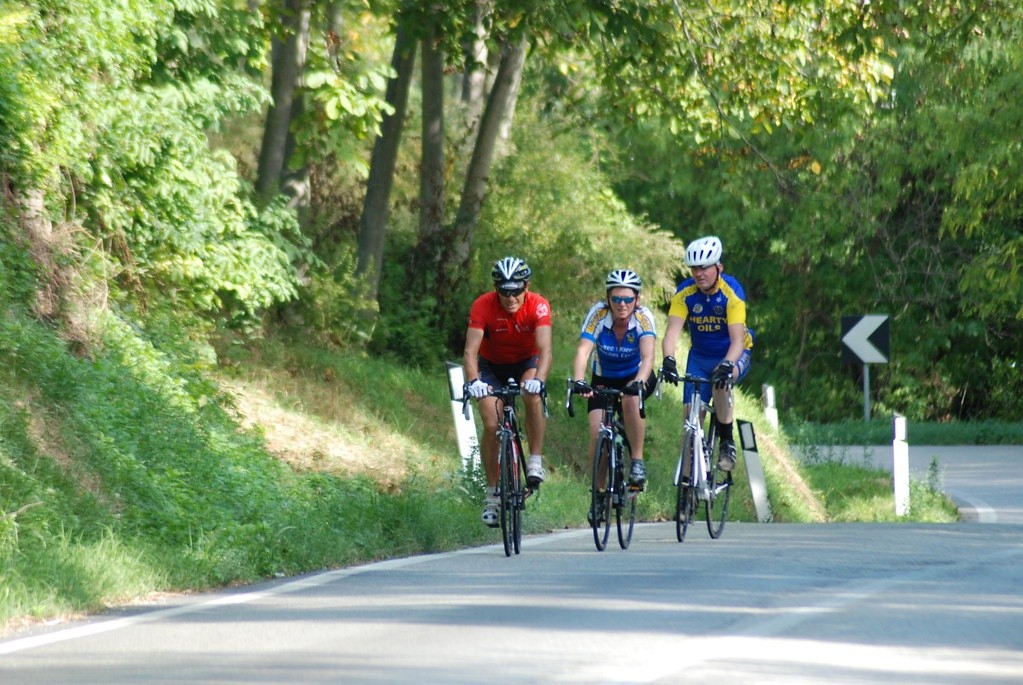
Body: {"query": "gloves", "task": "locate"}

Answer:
[662,356,678,387]
[520,378,542,395]
[468,380,493,398]
[711,361,733,392]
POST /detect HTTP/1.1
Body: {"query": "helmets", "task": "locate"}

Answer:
[605,270,642,296]
[491,257,531,290]
[685,236,722,268]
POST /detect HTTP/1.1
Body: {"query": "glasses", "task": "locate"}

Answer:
[497,285,524,297]
[609,295,635,303]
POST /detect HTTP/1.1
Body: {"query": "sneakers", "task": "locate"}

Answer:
[587,489,604,524]
[718,437,736,471]
[628,460,646,484]
[481,495,501,525]
[525,462,546,482]
[672,496,699,522]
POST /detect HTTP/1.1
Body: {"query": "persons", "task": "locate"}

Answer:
[661,236,756,524]
[462,256,552,525]
[574,270,656,525]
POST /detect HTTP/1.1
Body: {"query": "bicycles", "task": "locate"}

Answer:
[566,375,649,552]
[462,378,549,557]
[657,369,736,542]
